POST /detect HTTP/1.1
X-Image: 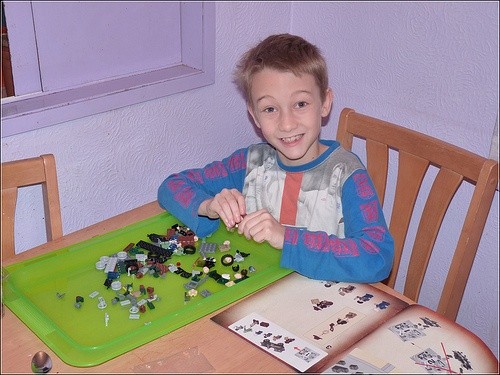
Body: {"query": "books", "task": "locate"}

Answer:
[210,274,498,373]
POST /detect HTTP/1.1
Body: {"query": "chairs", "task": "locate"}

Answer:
[336,108,498,323]
[0,154,62,261]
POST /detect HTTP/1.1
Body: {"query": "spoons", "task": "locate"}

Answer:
[31,351,52,373]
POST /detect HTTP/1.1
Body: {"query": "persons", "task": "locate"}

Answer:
[157,33,394,283]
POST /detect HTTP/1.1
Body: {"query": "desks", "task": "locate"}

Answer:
[0,200,416,375]
